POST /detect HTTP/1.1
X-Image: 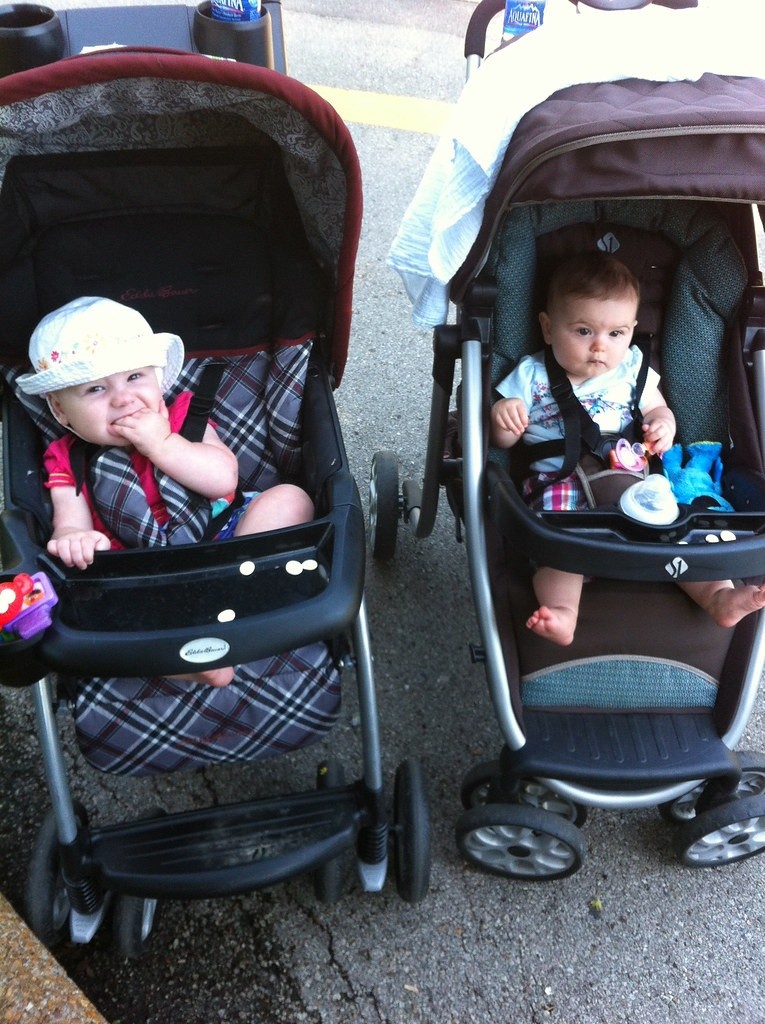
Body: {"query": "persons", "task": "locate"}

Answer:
[486,251,765,647]
[14,294,314,689]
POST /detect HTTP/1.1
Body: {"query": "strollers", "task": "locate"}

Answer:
[369,1,765,889]
[2,41,445,981]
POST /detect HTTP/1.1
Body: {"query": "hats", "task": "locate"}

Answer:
[13,296,185,399]
